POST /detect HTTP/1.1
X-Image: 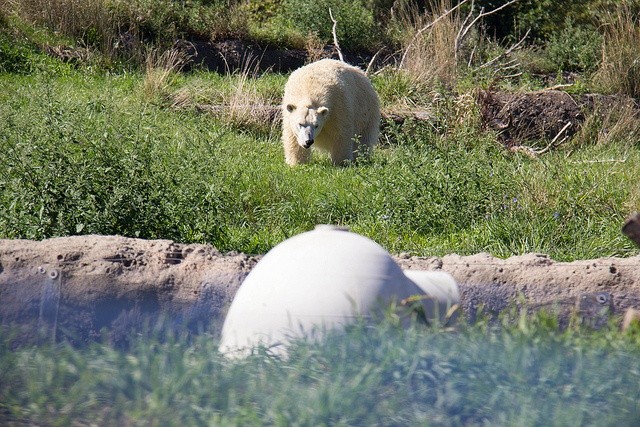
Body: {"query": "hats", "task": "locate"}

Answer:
[219,230,461,364]
